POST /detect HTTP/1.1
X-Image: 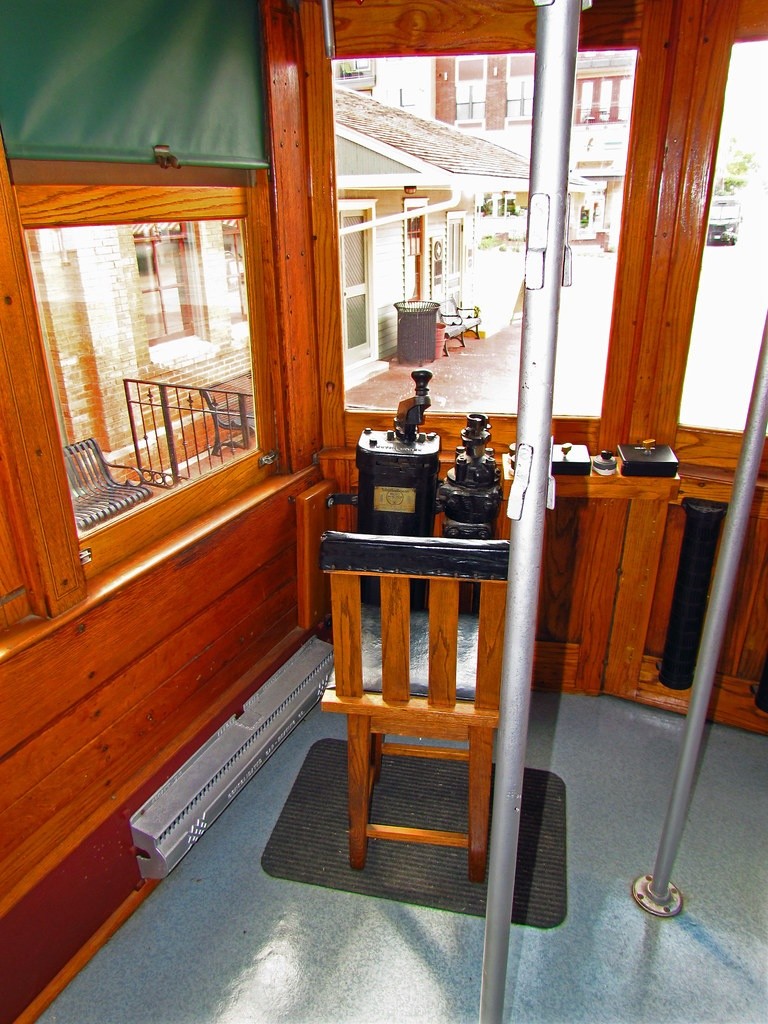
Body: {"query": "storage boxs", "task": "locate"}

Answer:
[394,299,442,365]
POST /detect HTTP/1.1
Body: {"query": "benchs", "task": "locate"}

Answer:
[61,437,154,531]
[433,298,481,357]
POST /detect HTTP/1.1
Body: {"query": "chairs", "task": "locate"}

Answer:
[316,528,514,884]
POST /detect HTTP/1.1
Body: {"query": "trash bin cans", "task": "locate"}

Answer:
[393,299,441,363]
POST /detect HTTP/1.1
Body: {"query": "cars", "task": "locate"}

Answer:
[706,198,745,247]
